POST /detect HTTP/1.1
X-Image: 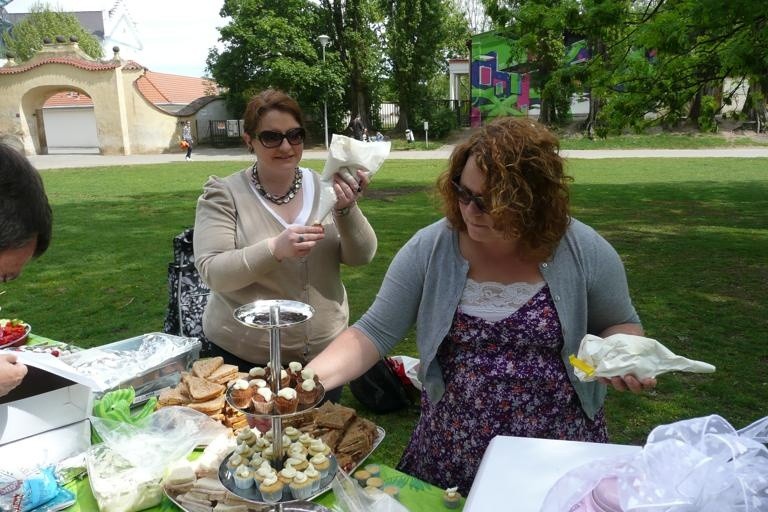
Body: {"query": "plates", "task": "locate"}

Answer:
[0,322,32,350]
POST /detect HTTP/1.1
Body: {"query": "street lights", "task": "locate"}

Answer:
[319,34,329,149]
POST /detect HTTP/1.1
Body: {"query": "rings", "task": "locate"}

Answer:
[300,234,304,242]
[353,186,362,192]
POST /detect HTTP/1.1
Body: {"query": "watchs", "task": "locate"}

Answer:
[334,206,349,217]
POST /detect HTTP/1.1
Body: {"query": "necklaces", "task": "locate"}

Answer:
[251,162,302,205]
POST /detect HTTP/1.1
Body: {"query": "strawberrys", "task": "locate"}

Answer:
[0,322,26,344]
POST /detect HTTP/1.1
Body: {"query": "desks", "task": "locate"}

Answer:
[0,328,472,511]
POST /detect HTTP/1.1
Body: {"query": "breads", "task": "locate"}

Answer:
[159,357,248,430]
[167,467,247,512]
[298,400,398,498]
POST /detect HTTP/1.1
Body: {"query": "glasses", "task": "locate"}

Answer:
[250,128,306,148]
[450,181,489,214]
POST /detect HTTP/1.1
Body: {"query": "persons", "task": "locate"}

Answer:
[192,89,378,407]
[182,120,194,161]
[0,143,52,397]
[304,117,658,497]
[349,113,369,142]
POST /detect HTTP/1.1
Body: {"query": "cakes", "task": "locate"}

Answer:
[311,221,325,239]
[444,487,461,507]
[227,362,332,502]
